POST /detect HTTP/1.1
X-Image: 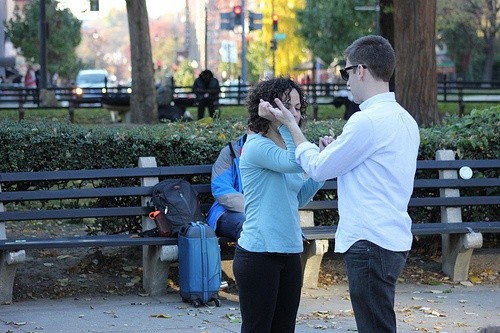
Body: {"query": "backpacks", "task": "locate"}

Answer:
[151,178,207,236]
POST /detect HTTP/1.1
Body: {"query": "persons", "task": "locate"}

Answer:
[24,64,37,104]
[232,77,335,333]
[206,131,246,241]
[192,70,220,119]
[267,35,419,333]
[34,64,40,88]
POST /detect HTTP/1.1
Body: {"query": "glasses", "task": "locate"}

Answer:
[339,64,368,81]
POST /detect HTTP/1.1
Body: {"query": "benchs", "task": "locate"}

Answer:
[0,151,500,306]
[0,75,500,125]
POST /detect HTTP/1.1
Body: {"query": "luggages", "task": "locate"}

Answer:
[177,221,223,308]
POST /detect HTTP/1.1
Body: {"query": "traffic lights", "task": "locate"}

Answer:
[271,40,278,50]
[233,7,242,33]
[273,16,278,21]
[219,13,234,29]
[249,11,263,31]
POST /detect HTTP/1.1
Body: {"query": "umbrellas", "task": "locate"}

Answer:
[293,59,326,70]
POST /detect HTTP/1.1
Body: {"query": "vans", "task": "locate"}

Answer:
[74,70,110,104]
